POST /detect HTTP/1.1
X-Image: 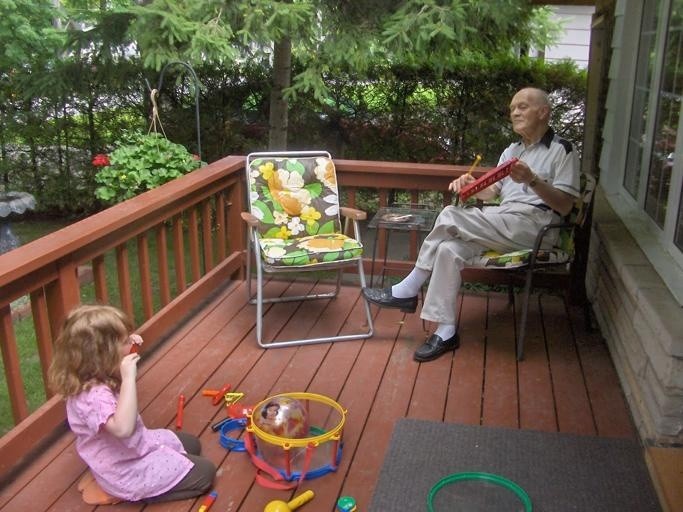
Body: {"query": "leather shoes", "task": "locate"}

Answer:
[412,328,461,362]
[360,285,419,314]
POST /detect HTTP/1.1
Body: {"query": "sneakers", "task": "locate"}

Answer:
[76,469,96,491]
[81,478,126,505]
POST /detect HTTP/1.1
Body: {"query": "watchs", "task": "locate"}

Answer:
[526,173,539,187]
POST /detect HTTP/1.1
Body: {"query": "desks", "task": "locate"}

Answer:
[362,201,439,333]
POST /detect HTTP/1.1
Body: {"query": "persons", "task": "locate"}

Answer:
[359,86,580,363]
[45,303,216,507]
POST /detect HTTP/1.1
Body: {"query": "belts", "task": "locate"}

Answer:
[528,203,561,217]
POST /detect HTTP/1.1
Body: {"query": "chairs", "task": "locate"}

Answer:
[241,150,375,349]
[455,171,597,363]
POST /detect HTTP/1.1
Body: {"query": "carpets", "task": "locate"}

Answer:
[367,415,664,512]
